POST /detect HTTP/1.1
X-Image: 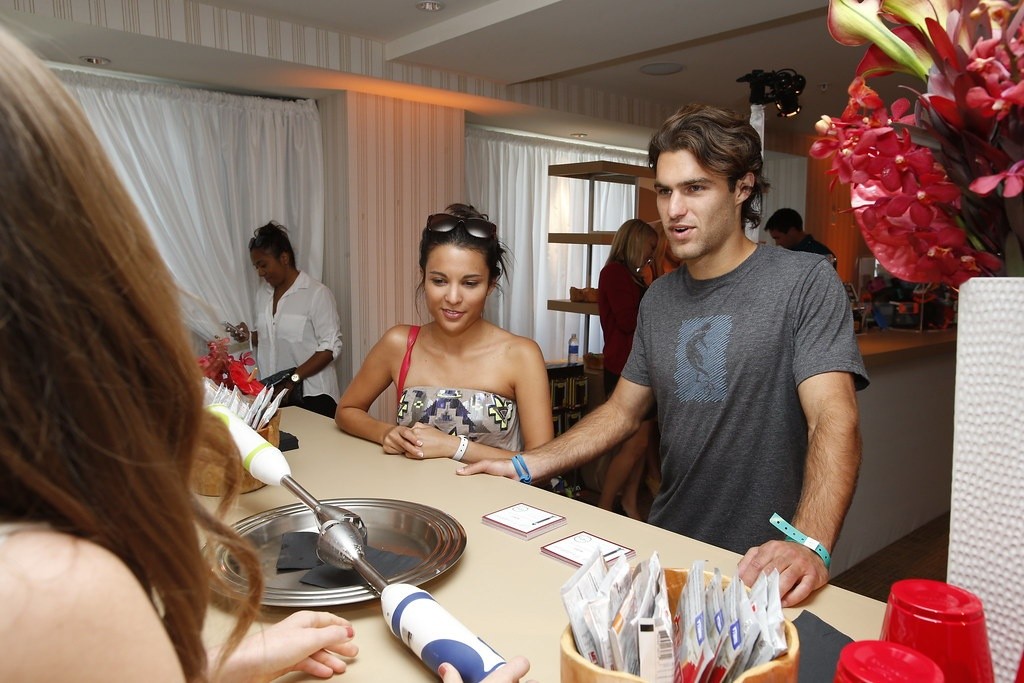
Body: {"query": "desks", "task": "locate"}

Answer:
[544,364,588,379]
[185,406,888,683]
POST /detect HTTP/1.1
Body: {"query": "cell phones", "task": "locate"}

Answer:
[220,321,247,338]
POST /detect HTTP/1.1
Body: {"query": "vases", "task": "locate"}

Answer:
[947,277,1023,683]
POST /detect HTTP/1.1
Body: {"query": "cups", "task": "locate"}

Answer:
[880,579,995,683]
[831,639,945,683]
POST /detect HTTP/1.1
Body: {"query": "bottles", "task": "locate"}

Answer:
[567,334,579,365]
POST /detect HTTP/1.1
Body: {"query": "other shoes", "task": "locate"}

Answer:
[615,501,628,517]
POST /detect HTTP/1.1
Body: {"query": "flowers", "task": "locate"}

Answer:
[198,335,269,396]
[808,0,1024,287]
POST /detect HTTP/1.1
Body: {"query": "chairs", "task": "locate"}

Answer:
[551,377,591,437]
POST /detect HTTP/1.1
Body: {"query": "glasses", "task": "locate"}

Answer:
[248,236,264,247]
[425,214,496,256]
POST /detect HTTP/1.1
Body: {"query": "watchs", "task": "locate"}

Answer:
[290,373,303,385]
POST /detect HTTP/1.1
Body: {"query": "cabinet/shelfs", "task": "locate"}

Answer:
[548,161,656,371]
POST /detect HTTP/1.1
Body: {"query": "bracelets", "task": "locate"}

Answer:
[452,434,469,460]
[769,512,831,568]
[511,454,532,484]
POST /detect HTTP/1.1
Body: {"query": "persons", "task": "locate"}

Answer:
[596,218,682,522]
[763,207,837,270]
[0,27,361,682]
[456,101,871,608]
[225,219,343,419]
[334,202,555,464]
